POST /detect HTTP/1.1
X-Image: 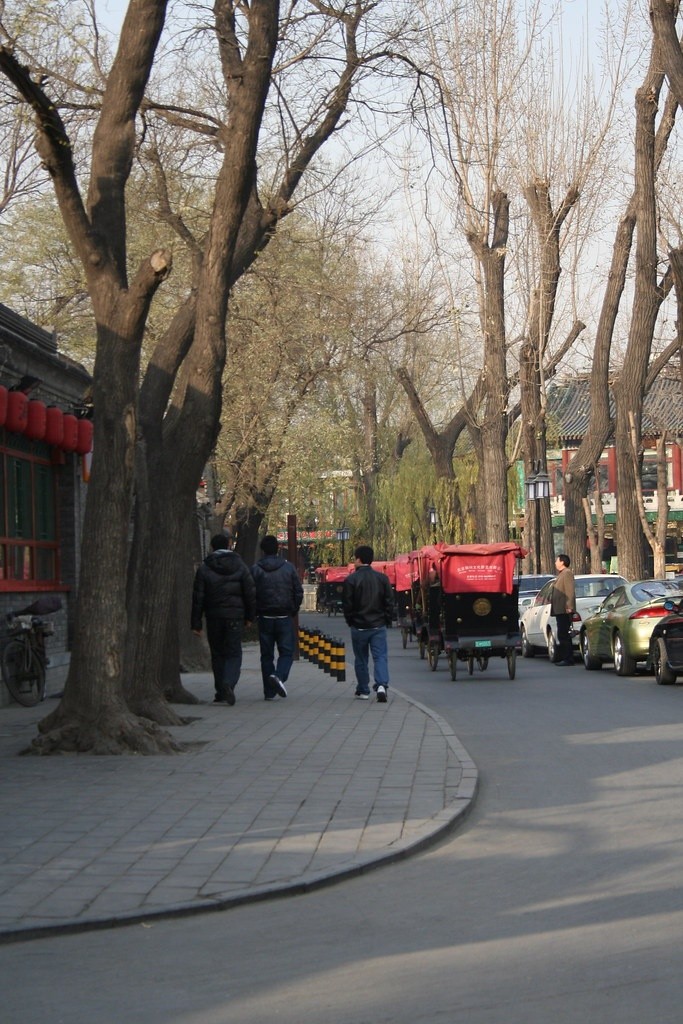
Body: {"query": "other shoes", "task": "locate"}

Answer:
[355,691,369,699]
[218,680,235,705]
[555,661,576,666]
[268,675,287,697]
[212,694,228,704]
[265,694,277,700]
[375,685,387,702]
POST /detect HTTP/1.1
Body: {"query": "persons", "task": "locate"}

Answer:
[248,536,303,700]
[341,546,394,702]
[191,535,249,705]
[550,554,576,666]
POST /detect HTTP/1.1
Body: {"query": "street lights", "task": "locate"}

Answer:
[430,506,439,545]
[336,526,351,567]
[524,459,553,574]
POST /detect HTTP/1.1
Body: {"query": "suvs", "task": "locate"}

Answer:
[518,573,633,664]
[512,574,557,627]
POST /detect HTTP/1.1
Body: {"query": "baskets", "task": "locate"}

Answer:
[5,614,32,637]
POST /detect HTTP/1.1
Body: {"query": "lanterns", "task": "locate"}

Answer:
[25,398,45,442]
[6,390,28,433]
[0,385,8,425]
[77,419,93,454]
[45,405,63,446]
[62,415,78,451]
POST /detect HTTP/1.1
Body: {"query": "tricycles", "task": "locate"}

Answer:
[315,544,521,683]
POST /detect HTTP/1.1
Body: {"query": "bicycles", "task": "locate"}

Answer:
[1,614,56,707]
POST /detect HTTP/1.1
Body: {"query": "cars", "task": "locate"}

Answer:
[578,578,683,686]
